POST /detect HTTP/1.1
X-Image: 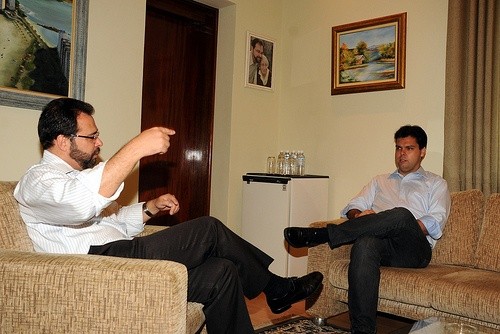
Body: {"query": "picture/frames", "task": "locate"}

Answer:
[331,12,407,95]
[244,31,276,92]
[0,0,90,111]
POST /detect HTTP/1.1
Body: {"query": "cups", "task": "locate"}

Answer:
[267,156,276,173]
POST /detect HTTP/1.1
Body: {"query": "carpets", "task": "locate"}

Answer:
[254,315,351,334]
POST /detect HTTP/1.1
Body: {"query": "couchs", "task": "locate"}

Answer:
[304,189,500,334]
[0,181,205,334]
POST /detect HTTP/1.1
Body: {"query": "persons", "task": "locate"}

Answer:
[11,95,325,334]
[256,55,272,88]
[248,38,265,84]
[283,123,452,334]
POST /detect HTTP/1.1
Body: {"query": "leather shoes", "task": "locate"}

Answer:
[267,270,323,314]
[284,226,328,248]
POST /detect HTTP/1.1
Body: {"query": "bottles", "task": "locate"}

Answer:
[284,150,291,174]
[291,151,305,175]
[277,150,285,174]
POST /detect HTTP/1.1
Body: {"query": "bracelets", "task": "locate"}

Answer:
[143,203,153,218]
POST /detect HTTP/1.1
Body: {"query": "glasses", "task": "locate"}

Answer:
[55,131,99,141]
